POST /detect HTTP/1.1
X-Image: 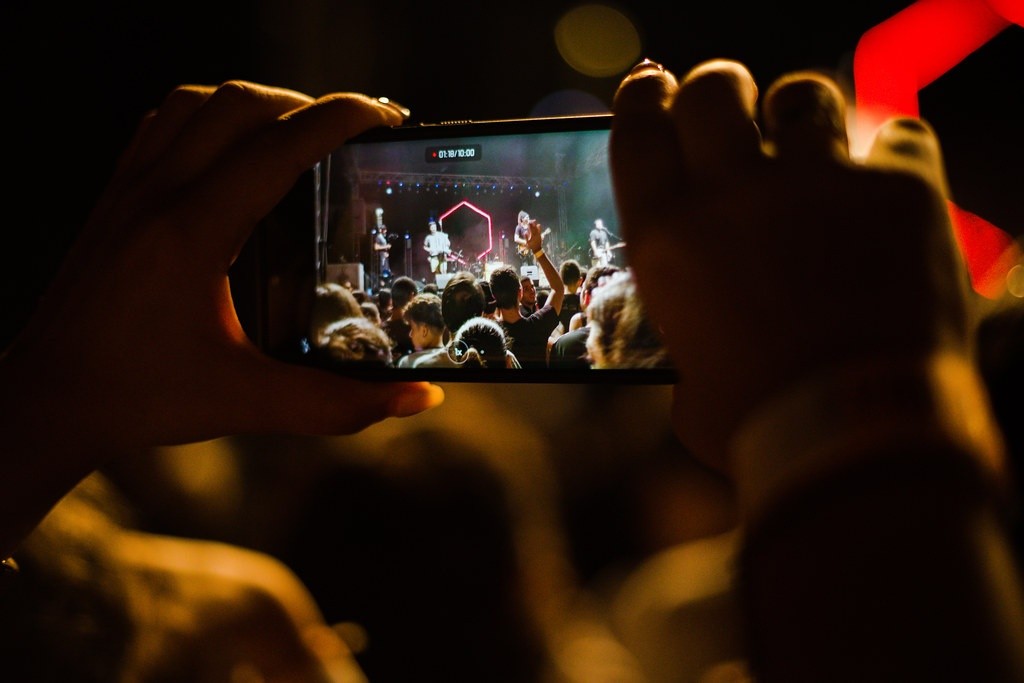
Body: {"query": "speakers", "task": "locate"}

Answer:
[352,198,366,236]
[433,273,455,291]
[521,265,539,288]
[485,261,504,283]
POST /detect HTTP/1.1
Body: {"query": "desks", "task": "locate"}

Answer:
[326,264,365,291]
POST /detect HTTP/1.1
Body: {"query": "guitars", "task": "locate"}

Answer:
[518,226,552,256]
[589,242,626,260]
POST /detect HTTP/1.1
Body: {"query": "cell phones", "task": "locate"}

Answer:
[256,113,678,386]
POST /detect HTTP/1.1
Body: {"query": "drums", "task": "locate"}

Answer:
[468,263,485,278]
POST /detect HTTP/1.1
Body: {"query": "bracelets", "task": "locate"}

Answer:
[723,358,1005,523]
[534,248,544,258]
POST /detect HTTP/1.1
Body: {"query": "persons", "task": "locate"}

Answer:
[1,54,1024,680]
[313,208,676,370]
